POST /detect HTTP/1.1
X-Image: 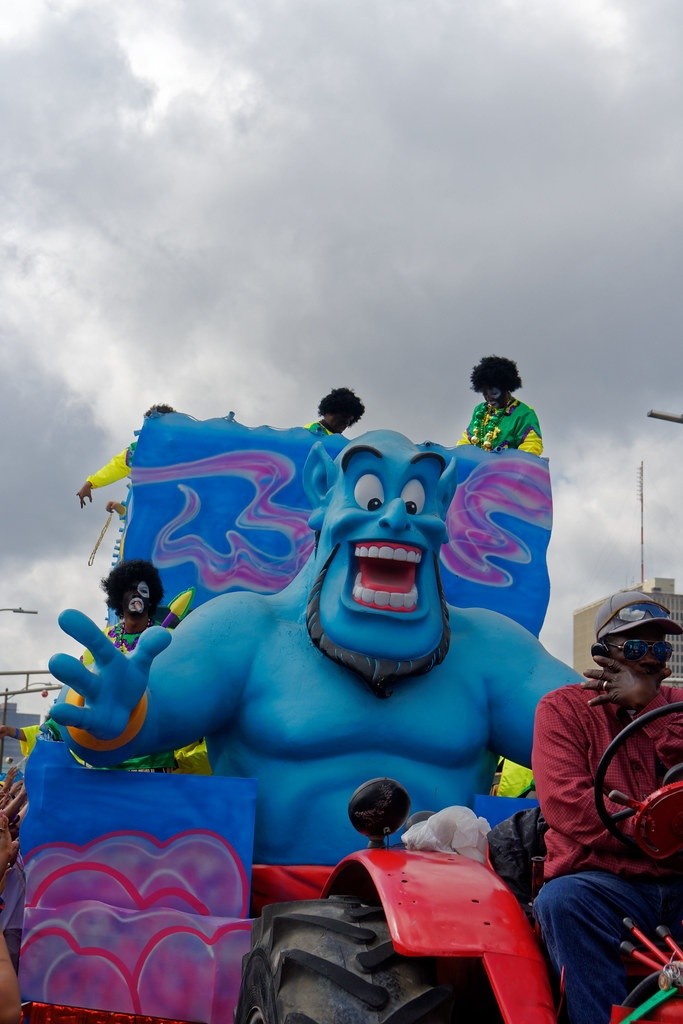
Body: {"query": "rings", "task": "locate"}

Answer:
[603,681,607,691]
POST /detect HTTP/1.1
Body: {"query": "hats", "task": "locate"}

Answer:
[595,591,683,642]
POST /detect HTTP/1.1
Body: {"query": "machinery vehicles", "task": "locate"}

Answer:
[232,702,683,1024]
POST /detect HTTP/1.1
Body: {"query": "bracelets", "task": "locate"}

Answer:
[16,728,19,739]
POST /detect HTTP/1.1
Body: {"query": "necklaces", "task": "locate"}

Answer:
[88,513,112,566]
[471,401,506,452]
[120,617,150,652]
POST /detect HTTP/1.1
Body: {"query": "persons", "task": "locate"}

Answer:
[0,725,42,757]
[533,590,683,1024]
[304,388,365,437]
[458,355,543,457]
[0,767,29,1024]
[45,557,180,770]
[105,500,126,522]
[75,404,174,508]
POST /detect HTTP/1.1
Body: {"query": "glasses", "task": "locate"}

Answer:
[610,603,670,622]
[605,640,673,662]
